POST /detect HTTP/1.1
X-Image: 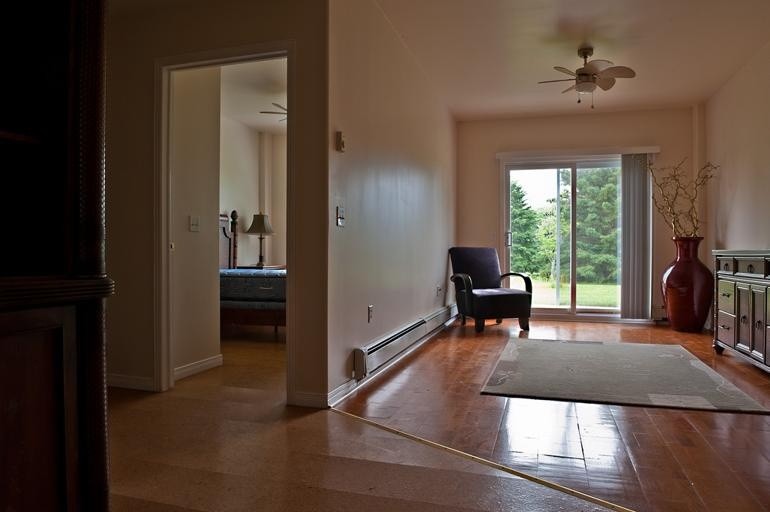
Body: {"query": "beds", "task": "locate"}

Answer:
[219,209,286,332]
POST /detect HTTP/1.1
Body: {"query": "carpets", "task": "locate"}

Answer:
[480,337,768,414]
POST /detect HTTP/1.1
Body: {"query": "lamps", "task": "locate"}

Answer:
[575,75,596,94]
[243,211,277,267]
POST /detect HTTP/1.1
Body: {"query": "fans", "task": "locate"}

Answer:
[536,47,636,110]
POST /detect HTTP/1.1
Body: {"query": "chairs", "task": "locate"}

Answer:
[448,246,533,332]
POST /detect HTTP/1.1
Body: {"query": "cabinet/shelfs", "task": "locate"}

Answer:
[712,249,770,374]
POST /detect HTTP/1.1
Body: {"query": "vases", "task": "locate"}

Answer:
[660,236,714,333]
